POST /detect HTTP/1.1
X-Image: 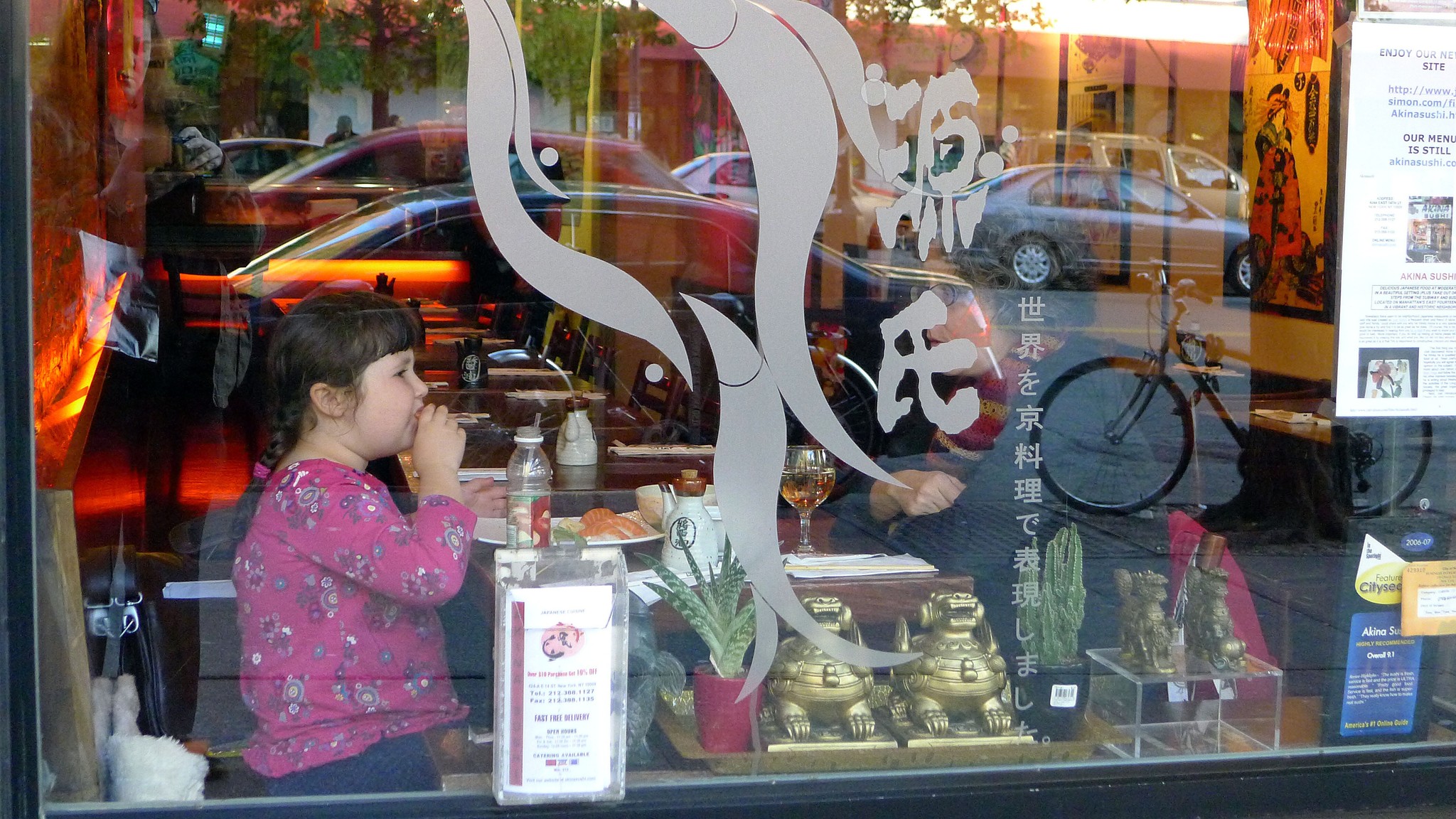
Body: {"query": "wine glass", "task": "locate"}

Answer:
[779,444,836,560]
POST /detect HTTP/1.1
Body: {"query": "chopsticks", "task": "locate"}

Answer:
[781,564,936,571]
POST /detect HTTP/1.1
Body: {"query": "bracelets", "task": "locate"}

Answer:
[96,189,123,221]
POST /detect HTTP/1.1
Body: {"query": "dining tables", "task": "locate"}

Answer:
[1198,397,1337,450]
[272,292,473,328]
[464,494,977,655]
[395,391,722,494]
[412,339,610,393]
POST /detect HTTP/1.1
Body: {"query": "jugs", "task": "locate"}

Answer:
[556,394,599,465]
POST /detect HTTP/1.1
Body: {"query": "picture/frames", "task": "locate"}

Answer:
[1221,0,1344,327]
[1053,34,1137,289]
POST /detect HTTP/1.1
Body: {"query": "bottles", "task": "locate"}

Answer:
[508,424,552,552]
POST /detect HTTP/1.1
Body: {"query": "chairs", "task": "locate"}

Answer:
[514,306,549,353]
[1165,510,1228,702]
[542,320,581,372]
[474,292,506,337]
[1194,378,1390,694]
[575,333,615,389]
[162,250,271,442]
[683,390,722,453]
[627,358,687,434]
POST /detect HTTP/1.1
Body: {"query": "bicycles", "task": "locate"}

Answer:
[1028,255,1434,519]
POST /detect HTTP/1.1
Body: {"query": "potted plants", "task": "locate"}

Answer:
[632,526,765,753]
[1014,521,1092,744]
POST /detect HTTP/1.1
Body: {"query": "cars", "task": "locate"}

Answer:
[220,138,320,182]
[253,122,688,189]
[223,182,1006,504]
[933,166,1254,289]
[667,152,912,247]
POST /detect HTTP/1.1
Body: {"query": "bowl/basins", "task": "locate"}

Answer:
[635,480,718,541]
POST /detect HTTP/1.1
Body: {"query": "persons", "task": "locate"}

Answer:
[227,288,517,798]
[27,0,268,785]
[385,114,402,128]
[323,113,361,147]
[828,188,1183,744]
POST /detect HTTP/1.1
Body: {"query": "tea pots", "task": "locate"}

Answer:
[454,334,488,389]
[659,468,720,570]
[373,272,396,297]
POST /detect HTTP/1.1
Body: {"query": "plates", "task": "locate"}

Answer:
[466,509,666,551]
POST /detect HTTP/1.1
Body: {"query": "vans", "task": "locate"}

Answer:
[1016,129,1250,225]
